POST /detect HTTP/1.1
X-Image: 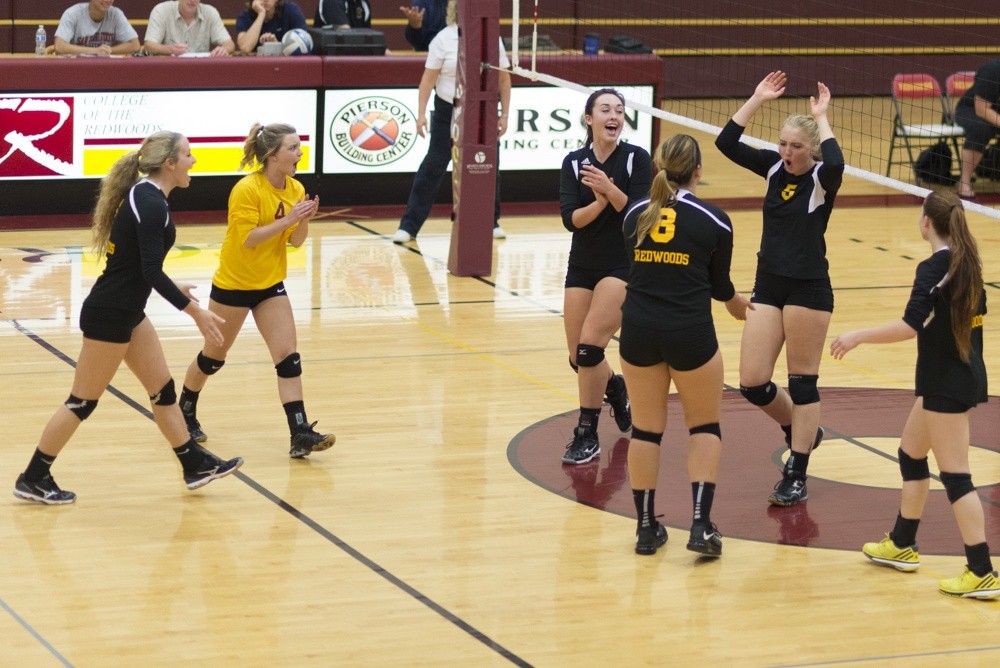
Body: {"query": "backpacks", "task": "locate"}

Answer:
[915,141,953,181]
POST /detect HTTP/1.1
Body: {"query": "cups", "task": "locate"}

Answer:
[584,31,600,55]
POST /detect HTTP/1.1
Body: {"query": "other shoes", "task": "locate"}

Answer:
[393,229,416,243]
[493,227,506,239]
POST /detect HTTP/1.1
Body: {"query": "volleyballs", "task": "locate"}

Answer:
[282,28,314,55]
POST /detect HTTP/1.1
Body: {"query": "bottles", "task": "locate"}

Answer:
[35,24,46,56]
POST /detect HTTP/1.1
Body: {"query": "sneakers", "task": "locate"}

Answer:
[785,425,824,456]
[634,514,668,555]
[181,410,208,442]
[862,530,920,573]
[686,521,723,555]
[767,467,809,507]
[13,470,77,505]
[561,426,601,465]
[288,420,336,457]
[183,454,244,490]
[603,374,632,433]
[936,562,1000,600]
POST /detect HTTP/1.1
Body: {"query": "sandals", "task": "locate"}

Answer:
[955,182,976,198]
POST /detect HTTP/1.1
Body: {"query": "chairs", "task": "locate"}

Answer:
[884,71,975,188]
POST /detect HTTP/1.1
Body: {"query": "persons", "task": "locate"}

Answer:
[619,134,755,557]
[11,131,244,507]
[54,0,140,56]
[399,0,447,52]
[829,188,1000,598]
[392,0,512,243]
[143,0,235,58]
[180,123,336,460]
[237,0,308,54]
[313,0,394,57]
[558,89,653,466]
[955,58,1000,195]
[715,69,845,507]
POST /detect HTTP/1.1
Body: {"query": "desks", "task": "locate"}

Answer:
[0,57,668,230]
